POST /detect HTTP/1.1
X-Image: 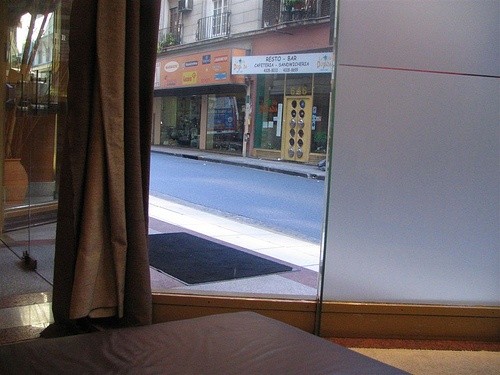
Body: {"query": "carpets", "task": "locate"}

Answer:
[349,348,500,375]
[148,232,292,286]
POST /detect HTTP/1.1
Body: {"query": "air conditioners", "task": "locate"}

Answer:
[177,0,193,12]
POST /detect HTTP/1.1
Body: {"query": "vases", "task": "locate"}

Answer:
[3,158,28,203]
